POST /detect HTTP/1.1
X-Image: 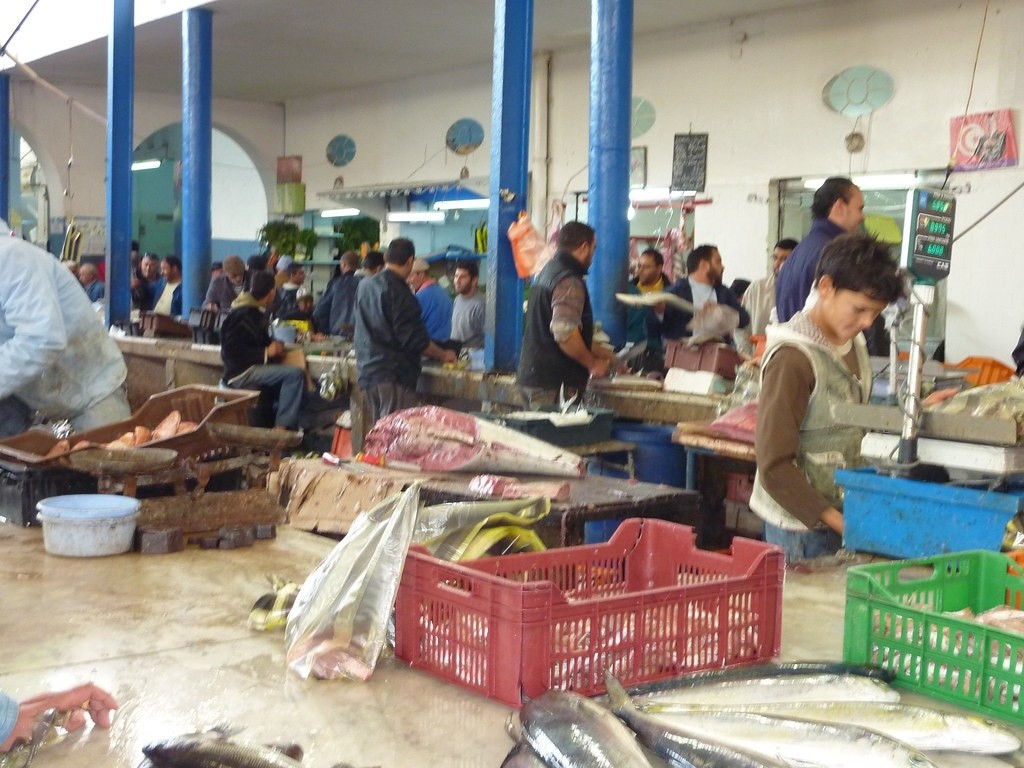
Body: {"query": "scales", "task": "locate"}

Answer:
[830,187,1024,490]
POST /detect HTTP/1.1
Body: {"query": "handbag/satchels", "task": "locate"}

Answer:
[279,492,421,680]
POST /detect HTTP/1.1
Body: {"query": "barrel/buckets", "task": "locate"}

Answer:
[582,423,692,545]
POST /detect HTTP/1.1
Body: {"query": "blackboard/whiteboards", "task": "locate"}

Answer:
[672,133,709,192]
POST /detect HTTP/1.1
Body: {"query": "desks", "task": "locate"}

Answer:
[279,457,703,590]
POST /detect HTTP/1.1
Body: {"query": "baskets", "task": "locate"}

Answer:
[398,516,787,709]
[842,551,1023,725]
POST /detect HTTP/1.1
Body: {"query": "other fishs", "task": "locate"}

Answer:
[43,411,200,460]
[498,661,1023,767]
[614,287,696,318]
[142,721,386,768]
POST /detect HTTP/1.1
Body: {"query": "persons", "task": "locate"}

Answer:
[974,115,1007,161]
[201,254,267,313]
[0,217,35,436]
[130,241,182,314]
[407,258,486,366]
[747,228,907,554]
[272,249,386,341]
[353,237,457,424]
[219,271,302,429]
[0,684,118,753]
[625,238,799,380]
[0,235,132,434]
[62,260,106,303]
[515,220,625,412]
[775,174,892,357]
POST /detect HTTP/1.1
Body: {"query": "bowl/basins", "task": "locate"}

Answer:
[36,511,142,557]
[36,494,143,518]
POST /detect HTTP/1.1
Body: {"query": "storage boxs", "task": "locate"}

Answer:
[115,307,229,347]
[395,517,786,714]
[331,411,351,458]
[834,468,1024,567]
[0,384,261,526]
[842,549,1024,725]
[664,340,745,397]
[721,475,766,535]
[945,358,1016,388]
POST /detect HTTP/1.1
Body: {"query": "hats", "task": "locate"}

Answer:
[223,257,244,275]
